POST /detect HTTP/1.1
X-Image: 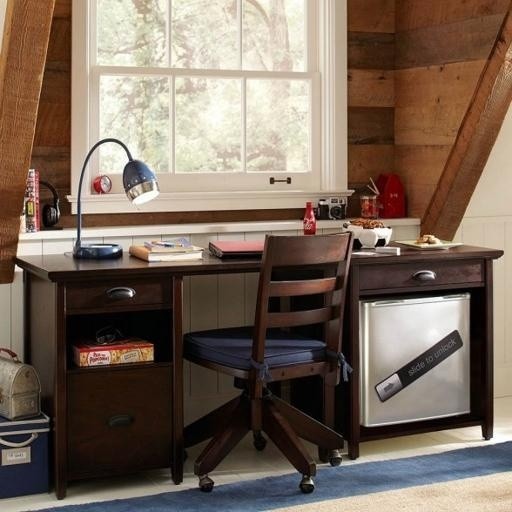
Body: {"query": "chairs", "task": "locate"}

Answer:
[181,230,357,495]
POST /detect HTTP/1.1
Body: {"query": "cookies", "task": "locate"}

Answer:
[415,234,440,244]
[351,218,384,229]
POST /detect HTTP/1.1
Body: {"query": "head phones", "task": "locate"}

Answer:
[39,180,61,227]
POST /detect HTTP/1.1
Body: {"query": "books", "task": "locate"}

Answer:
[25,168,41,232]
[144,237,193,252]
[128,242,206,262]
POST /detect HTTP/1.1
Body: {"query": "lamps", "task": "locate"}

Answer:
[69,133,162,262]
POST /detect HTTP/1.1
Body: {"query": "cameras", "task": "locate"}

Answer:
[318,197,346,219]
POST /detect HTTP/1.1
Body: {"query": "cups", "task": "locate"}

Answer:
[359,194,380,220]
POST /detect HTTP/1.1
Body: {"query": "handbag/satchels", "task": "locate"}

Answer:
[0,348,42,421]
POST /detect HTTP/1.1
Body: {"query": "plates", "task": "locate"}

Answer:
[395,239,463,250]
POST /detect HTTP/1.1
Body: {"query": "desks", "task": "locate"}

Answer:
[12,238,506,502]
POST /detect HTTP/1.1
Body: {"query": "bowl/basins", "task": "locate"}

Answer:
[341,227,392,247]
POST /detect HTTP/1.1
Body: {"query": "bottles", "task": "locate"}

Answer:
[303,202,316,236]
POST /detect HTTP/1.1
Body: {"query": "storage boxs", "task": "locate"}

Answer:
[0,411,53,502]
